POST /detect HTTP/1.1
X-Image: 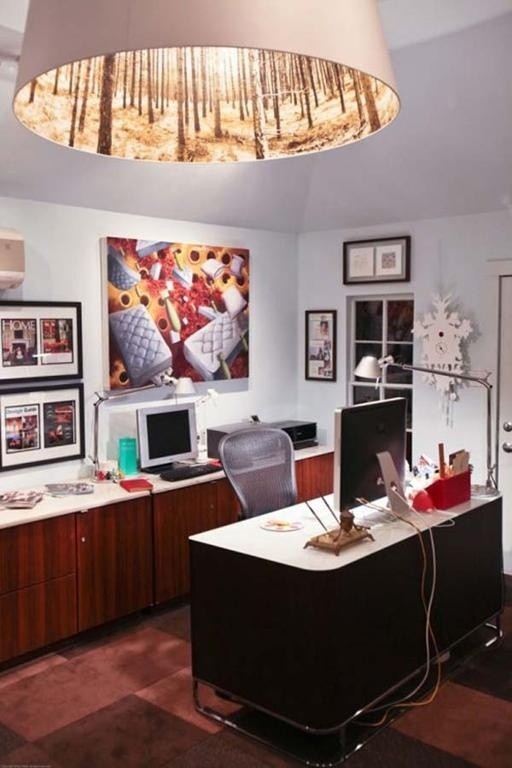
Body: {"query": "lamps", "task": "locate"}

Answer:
[10,1,402,167]
[353,353,499,499]
[86,374,198,483]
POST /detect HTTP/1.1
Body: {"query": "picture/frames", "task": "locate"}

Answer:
[342,235,411,284]
[304,309,336,382]
[0,299,84,384]
[0,386,86,473]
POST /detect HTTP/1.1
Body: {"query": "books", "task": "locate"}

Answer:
[120,478,154,493]
[5,494,37,509]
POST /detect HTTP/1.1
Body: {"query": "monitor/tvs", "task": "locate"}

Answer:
[136,402,199,474]
[331,397,407,531]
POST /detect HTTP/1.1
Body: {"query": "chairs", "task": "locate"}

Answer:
[218,426,298,523]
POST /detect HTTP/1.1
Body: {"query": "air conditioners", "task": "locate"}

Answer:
[0,227,26,292]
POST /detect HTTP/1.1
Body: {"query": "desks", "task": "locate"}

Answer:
[187,482,502,767]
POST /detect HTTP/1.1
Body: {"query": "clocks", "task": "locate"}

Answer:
[409,292,475,429]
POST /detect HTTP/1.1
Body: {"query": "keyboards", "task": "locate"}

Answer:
[160,463,222,481]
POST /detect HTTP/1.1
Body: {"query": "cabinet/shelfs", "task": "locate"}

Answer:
[294,461,303,505]
[0,513,79,662]
[77,494,154,636]
[217,477,243,531]
[303,450,335,503]
[155,478,218,605]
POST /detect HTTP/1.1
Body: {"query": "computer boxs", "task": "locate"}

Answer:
[206,422,281,459]
